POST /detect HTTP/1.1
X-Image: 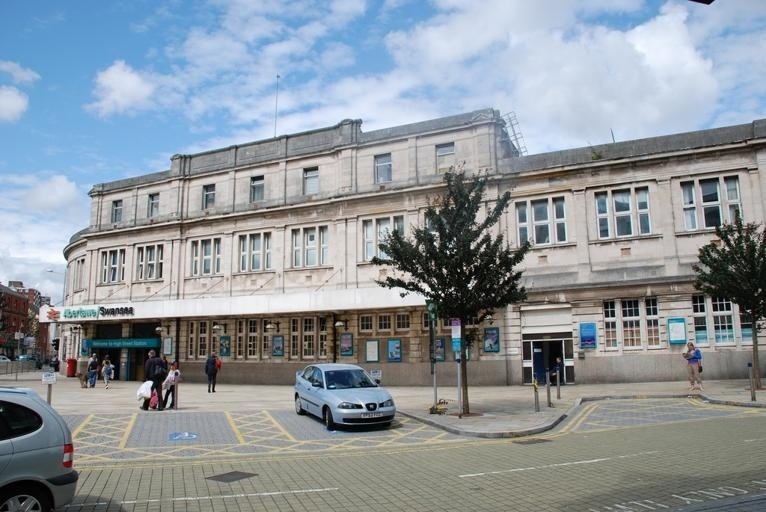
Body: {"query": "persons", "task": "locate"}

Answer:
[87,357,100,387]
[682,341,705,392]
[551,357,565,385]
[204,351,220,393]
[100,353,112,383]
[85,352,101,387]
[139,350,167,411]
[101,360,111,389]
[161,361,181,409]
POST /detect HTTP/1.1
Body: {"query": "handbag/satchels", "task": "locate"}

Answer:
[215,357,222,370]
[110,370,115,380]
[697,365,703,373]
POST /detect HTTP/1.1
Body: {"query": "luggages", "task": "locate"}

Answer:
[80,371,88,388]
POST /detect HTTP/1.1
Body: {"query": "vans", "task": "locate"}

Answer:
[0,355,11,363]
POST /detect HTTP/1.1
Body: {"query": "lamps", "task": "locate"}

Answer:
[266,320,279,333]
[335,318,347,331]
[74,327,87,336]
[485,314,496,324]
[212,323,227,333]
[155,325,170,335]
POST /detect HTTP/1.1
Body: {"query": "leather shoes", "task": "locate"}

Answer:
[140,406,148,410]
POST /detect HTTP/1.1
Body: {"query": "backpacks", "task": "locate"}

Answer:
[105,366,112,375]
[153,362,168,382]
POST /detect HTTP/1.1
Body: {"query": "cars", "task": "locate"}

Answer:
[293,362,396,430]
[17,355,42,370]
[1,386,79,511]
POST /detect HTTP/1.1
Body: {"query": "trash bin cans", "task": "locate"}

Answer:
[66,359,77,377]
